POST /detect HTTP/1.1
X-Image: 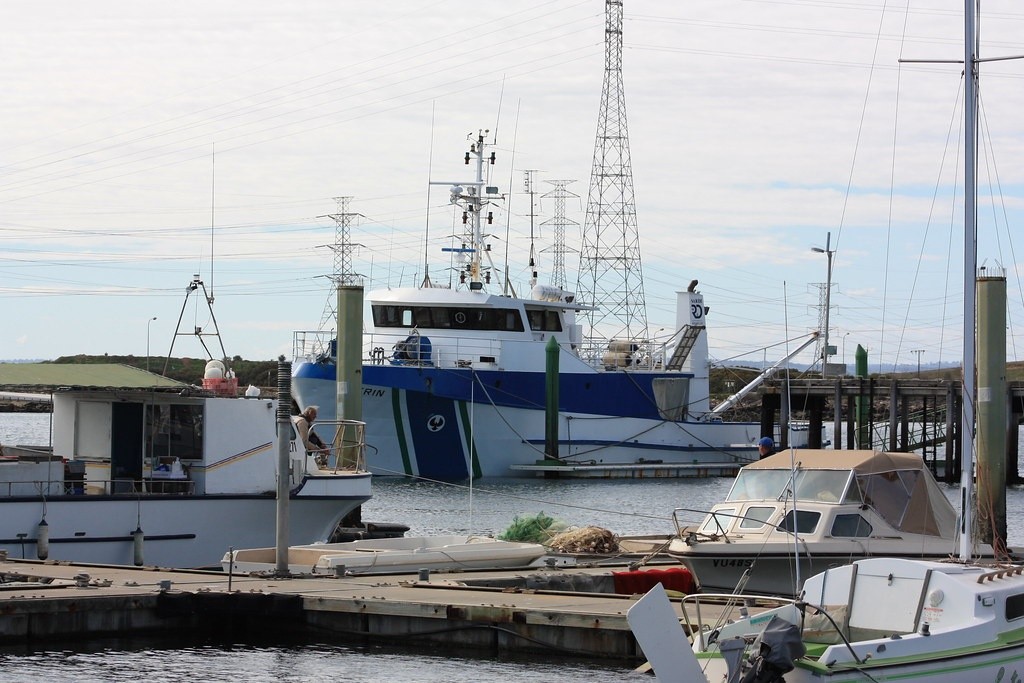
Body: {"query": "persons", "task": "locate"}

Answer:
[294,406,330,456]
[758,437,776,460]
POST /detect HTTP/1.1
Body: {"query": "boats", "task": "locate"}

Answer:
[0,141,378,571]
[220,380,544,579]
[626,281,1024,683]
[291,71,832,482]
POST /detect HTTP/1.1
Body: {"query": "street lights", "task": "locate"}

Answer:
[147,317,157,370]
[842,332,849,365]
